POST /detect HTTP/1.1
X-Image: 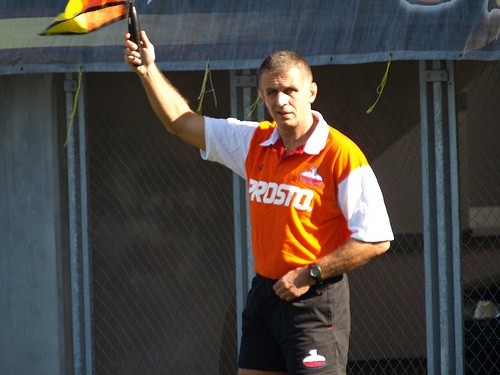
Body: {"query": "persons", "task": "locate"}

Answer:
[122,30,395,375]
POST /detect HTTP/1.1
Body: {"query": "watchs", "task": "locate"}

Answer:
[308,262,324,289]
